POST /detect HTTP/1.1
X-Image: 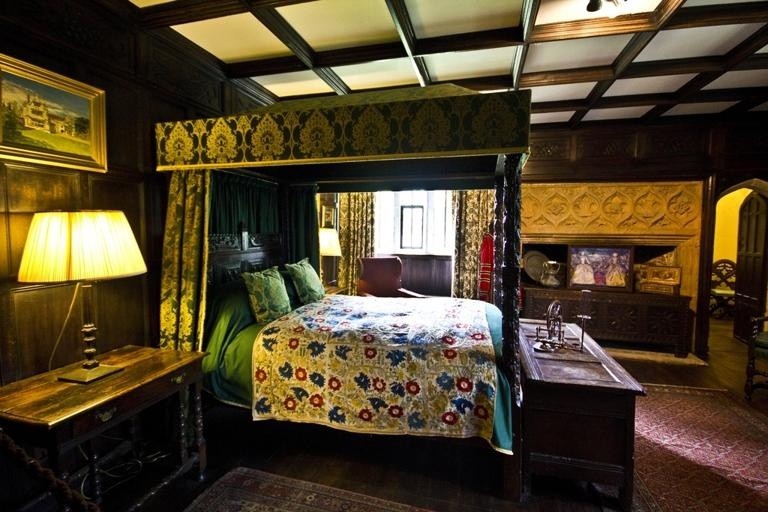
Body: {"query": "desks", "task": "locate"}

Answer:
[518,317,648,512]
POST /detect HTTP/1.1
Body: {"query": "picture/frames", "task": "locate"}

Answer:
[322,205,334,227]
[567,245,635,294]
[646,266,682,285]
[0,53,109,177]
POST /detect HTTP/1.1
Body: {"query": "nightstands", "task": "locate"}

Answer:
[326,283,349,296]
[0,342,211,512]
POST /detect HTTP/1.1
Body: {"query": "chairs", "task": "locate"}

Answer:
[356,256,425,298]
[744,314,768,401]
[710,259,737,319]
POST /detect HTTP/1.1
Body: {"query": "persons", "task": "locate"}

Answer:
[606,252,626,287]
[572,251,595,285]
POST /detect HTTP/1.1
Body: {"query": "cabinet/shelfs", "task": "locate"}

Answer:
[520,180,704,358]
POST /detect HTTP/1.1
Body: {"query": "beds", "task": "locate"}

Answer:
[147,89,548,503]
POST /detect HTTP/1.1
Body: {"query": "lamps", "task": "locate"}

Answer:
[17,209,148,383]
[317,227,343,291]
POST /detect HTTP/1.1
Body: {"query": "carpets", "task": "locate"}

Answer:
[633,381,768,512]
[182,464,435,512]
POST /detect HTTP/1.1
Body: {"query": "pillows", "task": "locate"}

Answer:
[283,256,325,304]
[238,264,293,331]
